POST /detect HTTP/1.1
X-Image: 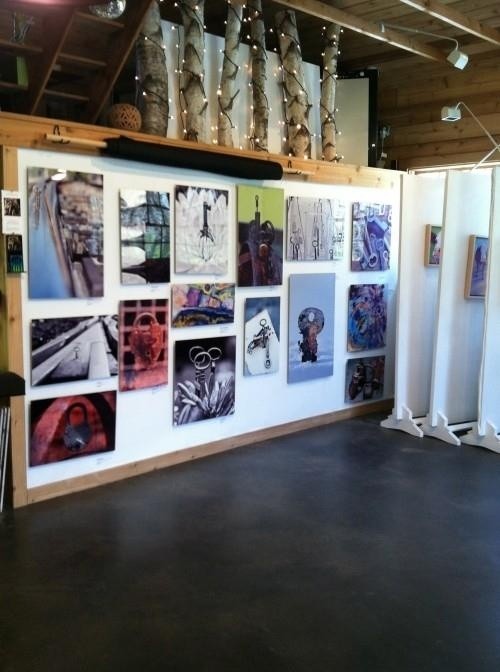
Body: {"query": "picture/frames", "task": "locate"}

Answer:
[424,225,441,267]
[464,235,489,299]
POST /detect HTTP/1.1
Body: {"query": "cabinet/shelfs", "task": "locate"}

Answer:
[0,0,155,124]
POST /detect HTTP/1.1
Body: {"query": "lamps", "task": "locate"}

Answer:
[379,21,468,70]
[441,101,500,169]
